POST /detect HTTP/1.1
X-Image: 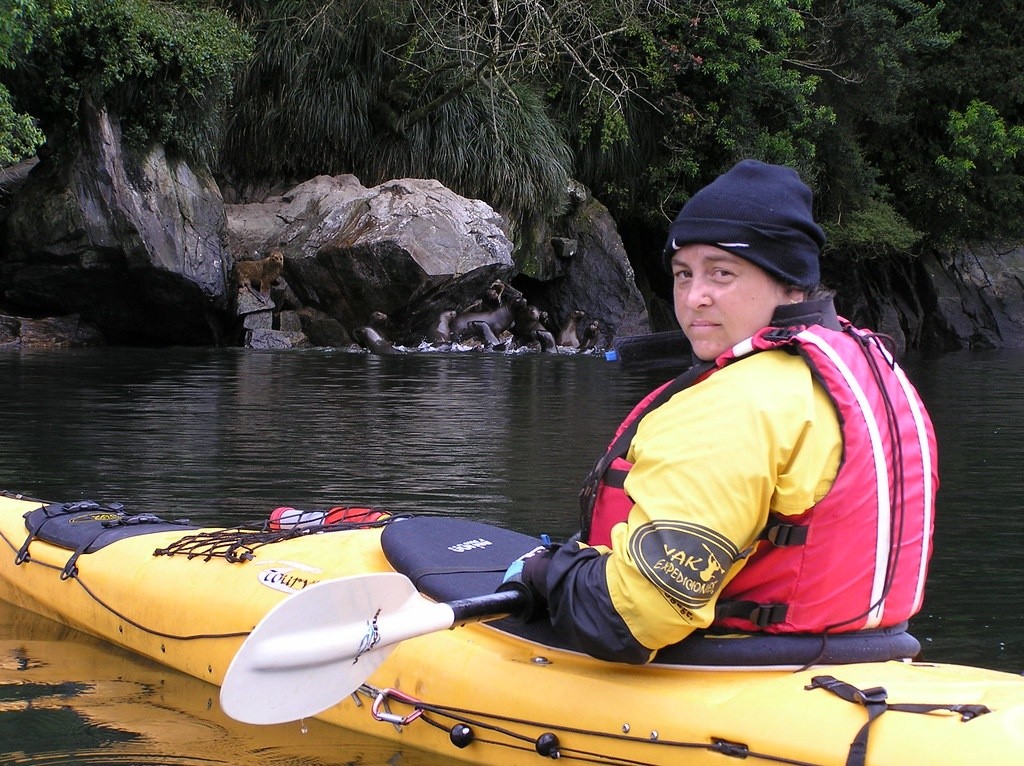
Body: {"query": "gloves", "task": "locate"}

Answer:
[520,553,542,586]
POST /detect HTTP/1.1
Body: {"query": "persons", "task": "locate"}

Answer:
[505,159,936,664]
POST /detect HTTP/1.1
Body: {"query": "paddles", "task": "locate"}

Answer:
[219,572,548,725]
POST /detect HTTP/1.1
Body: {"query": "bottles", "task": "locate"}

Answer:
[268,505,391,532]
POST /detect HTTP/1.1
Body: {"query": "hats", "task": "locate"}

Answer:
[661,159,826,292]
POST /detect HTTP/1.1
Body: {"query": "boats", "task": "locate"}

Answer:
[0,497,1024,764]
